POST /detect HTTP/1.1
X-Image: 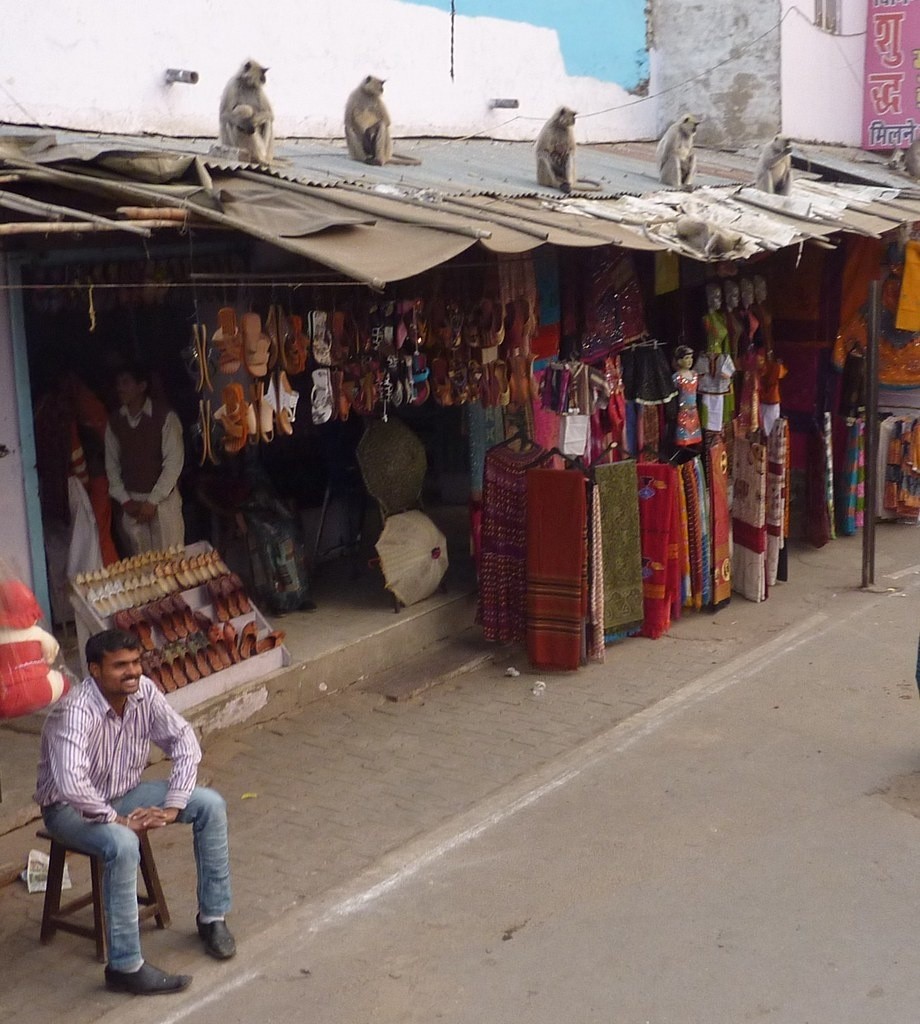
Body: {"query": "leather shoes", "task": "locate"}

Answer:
[104,962,193,997]
[196,913,235,958]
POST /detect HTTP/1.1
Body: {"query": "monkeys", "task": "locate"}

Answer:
[535,106,604,194]
[903,138,920,178]
[343,75,420,166]
[656,114,702,193]
[216,59,274,166]
[755,135,793,196]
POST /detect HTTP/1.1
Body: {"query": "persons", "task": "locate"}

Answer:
[233,443,318,618]
[701,274,785,438]
[104,365,183,554]
[670,345,705,446]
[34,629,235,995]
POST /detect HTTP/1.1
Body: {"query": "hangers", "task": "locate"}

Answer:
[626,445,678,468]
[525,448,585,471]
[710,434,726,448]
[504,419,540,450]
[669,447,702,461]
[588,441,635,470]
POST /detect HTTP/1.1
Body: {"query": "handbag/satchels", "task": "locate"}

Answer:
[533,363,589,457]
[76,424,104,476]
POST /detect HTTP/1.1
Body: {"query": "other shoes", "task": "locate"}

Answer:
[273,610,285,616]
[29,253,247,310]
[141,655,166,694]
[151,648,177,692]
[75,544,225,618]
[187,636,211,676]
[170,644,200,681]
[356,289,534,414]
[297,602,318,612]
[161,647,187,687]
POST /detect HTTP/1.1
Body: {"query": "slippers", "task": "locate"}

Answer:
[129,609,153,650]
[240,621,258,658]
[208,626,232,667]
[229,574,251,613]
[258,631,286,652]
[159,600,188,637]
[220,577,240,616]
[207,581,229,621]
[224,623,241,663]
[197,631,223,671]
[209,305,355,449]
[145,605,178,641]
[116,612,145,653]
[172,593,198,633]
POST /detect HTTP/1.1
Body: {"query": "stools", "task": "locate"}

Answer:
[36,827,173,965]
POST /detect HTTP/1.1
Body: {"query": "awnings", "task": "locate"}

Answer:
[4,129,920,292]
